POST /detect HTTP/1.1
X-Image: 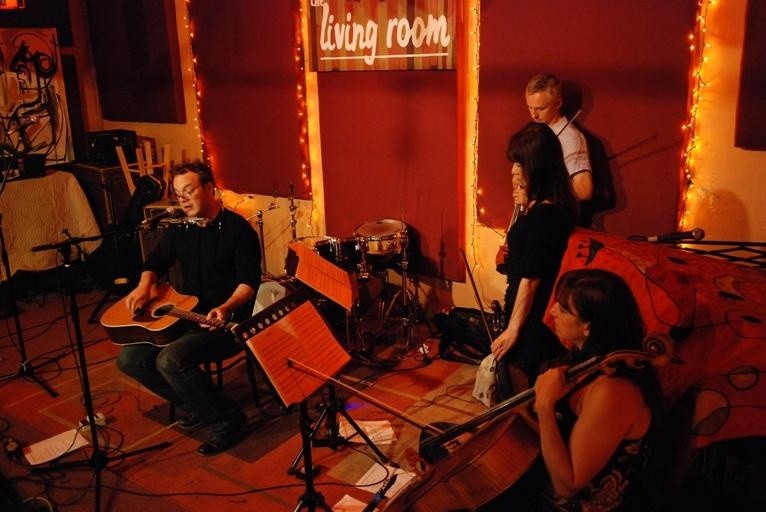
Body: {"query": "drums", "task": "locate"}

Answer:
[338,238,372,277]
[289,235,336,264]
[350,267,388,311]
[355,219,408,254]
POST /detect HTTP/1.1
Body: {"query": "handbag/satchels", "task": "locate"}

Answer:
[436,305,501,366]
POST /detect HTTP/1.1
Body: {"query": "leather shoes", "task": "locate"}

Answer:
[177,412,203,433]
[195,425,247,457]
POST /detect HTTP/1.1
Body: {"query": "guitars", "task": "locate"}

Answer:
[100,283,238,347]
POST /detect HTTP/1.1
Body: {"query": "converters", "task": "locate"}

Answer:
[79,413,105,432]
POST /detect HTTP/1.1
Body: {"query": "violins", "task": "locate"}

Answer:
[485,301,527,403]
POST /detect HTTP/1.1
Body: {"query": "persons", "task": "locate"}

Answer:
[490,122,583,390]
[114,160,263,455]
[510,71,594,210]
[441,267,664,512]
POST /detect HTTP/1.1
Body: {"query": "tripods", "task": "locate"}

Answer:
[29,223,169,512]
[293,402,334,512]
[287,383,388,475]
[0,133,67,397]
[375,241,435,365]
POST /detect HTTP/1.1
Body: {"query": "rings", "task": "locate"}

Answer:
[497,343,503,348]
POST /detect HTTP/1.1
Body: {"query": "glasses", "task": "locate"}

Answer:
[170,184,199,201]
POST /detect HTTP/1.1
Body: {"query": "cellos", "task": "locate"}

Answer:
[382,332,674,510]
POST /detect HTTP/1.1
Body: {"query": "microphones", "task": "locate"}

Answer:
[647,228,705,242]
[141,207,177,225]
[7,114,40,134]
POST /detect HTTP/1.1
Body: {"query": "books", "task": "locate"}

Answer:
[295,243,352,312]
[244,300,352,409]
